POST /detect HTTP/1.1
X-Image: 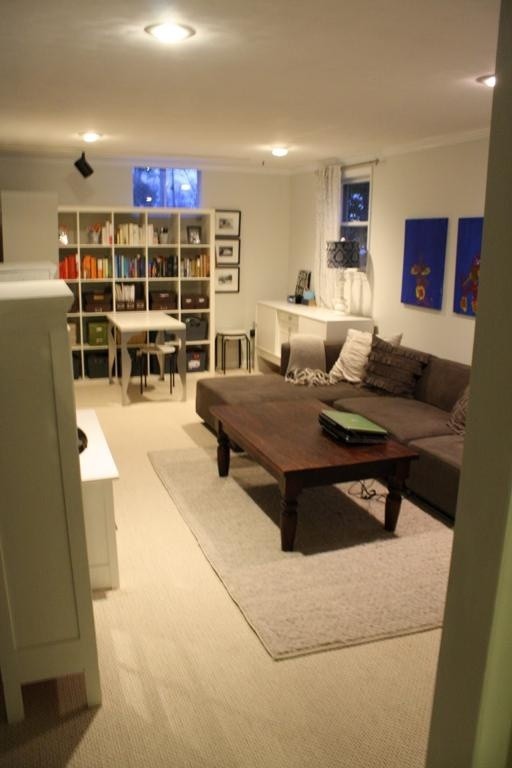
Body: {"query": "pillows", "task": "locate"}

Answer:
[360,333,432,398]
[328,327,404,383]
[446,384,470,433]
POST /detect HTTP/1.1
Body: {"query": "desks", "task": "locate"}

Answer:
[105,310,188,405]
[254,298,373,367]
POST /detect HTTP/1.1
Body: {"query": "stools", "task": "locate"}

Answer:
[215,329,252,374]
[137,344,176,394]
[223,335,248,374]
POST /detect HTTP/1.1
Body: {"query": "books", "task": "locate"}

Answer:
[59,221,210,279]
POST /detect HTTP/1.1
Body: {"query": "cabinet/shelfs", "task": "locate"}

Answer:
[73,408,123,593]
[56,204,216,383]
[1,259,105,725]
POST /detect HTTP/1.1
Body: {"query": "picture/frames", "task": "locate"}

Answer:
[216,265,240,293]
[215,209,241,237]
[216,237,241,264]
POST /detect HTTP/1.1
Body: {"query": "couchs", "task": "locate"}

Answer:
[195,338,472,520]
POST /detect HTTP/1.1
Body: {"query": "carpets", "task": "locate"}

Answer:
[146,444,456,662]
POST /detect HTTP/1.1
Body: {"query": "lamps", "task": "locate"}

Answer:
[73,151,95,179]
[326,240,360,312]
